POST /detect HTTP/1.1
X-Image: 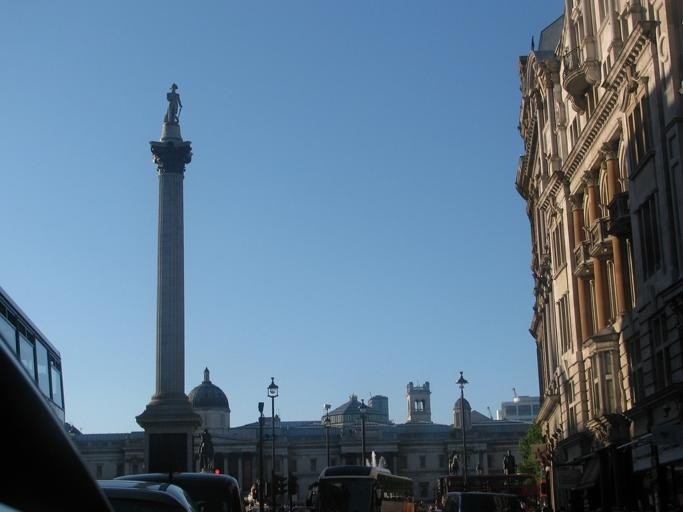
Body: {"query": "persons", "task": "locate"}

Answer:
[199,429,211,453]
[502,448,516,474]
[163,84,182,126]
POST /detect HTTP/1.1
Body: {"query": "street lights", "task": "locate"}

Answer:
[266,377,281,511]
[455,370,472,493]
[354,399,370,466]
[319,404,333,469]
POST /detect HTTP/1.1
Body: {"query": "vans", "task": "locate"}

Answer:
[439,491,521,512]
[110,471,243,512]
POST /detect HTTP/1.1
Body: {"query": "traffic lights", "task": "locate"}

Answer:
[213,468,221,474]
[271,471,288,496]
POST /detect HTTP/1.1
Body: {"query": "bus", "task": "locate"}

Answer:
[437,474,538,500]
[306,464,414,512]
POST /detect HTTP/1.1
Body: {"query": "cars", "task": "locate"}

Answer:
[0,335,117,512]
[95,479,195,512]
